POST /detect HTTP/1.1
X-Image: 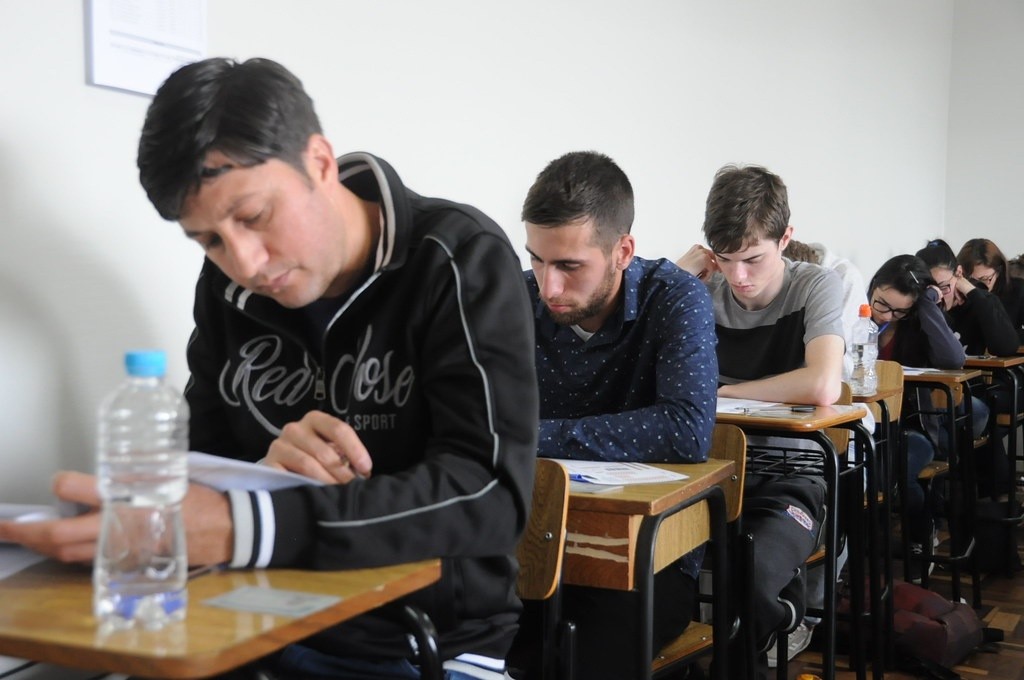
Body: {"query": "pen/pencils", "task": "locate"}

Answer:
[878,321,892,336]
[737,404,817,413]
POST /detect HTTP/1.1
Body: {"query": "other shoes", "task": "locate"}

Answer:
[708,646,759,680]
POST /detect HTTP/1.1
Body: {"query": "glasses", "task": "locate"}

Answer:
[979,270,999,286]
[937,271,956,295]
[873,289,915,319]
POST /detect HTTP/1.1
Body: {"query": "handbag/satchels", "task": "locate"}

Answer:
[838,569,983,671]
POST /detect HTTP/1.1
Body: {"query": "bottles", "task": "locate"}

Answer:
[846,303,880,398]
[92,348,191,633]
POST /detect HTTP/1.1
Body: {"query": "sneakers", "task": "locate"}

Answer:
[906,517,936,583]
[767,618,817,667]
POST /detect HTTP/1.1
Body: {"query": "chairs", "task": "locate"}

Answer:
[997,345,1024,502]
[651,423,760,680]
[777,382,853,680]
[919,382,983,603]
[849,361,904,672]
[514,458,579,680]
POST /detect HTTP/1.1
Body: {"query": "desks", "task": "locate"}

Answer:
[0,356,1024,680]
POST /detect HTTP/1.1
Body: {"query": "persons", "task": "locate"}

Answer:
[677,168,1024,667]
[519,149,720,680]
[1,53,544,680]
